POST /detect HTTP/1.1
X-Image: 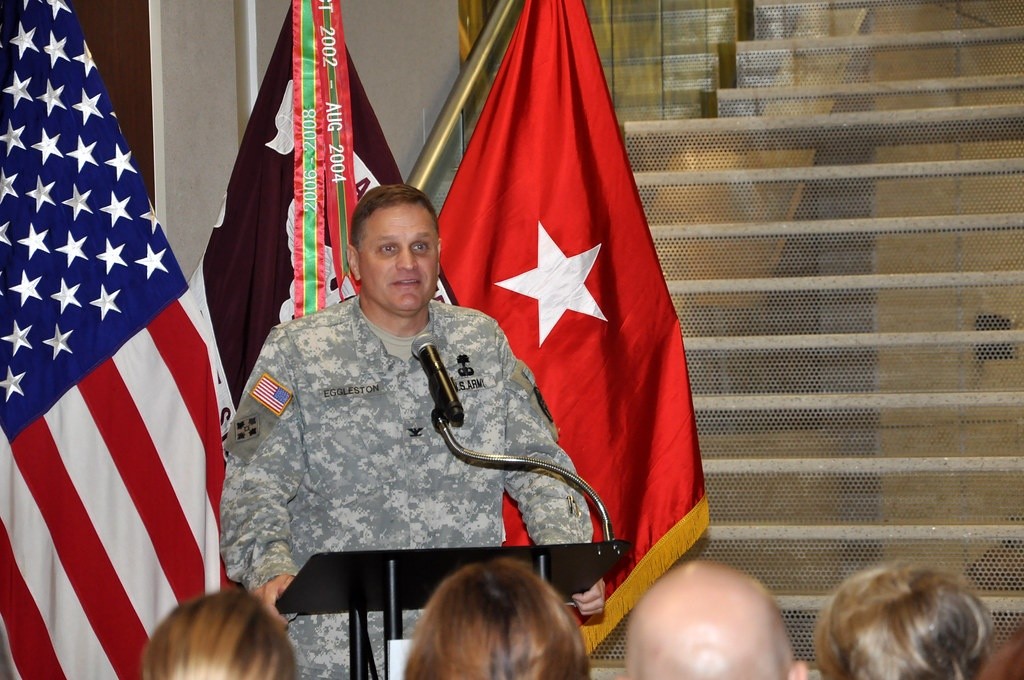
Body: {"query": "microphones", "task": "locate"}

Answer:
[410,333,464,423]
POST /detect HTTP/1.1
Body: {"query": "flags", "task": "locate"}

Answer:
[187,0,460,466]
[437,0,710,655]
[0,0,242,680]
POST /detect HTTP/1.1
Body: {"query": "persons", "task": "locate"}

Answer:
[220,184,605,680]
[143,591,296,680]
[618,561,807,680]
[814,540,1024,680]
[405,557,590,680]
